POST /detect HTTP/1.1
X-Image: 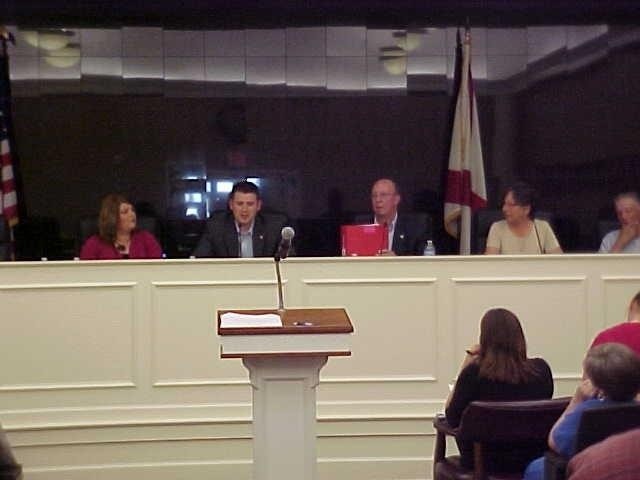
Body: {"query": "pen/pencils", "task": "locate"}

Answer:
[292,322,313,326]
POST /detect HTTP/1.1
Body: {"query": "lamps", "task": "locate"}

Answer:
[39,44,82,69]
[375,30,433,76]
[22,26,77,49]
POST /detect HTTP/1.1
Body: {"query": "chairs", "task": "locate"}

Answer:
[546,400,640,480]
[429,391,577,479]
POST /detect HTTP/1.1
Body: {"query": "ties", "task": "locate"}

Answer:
[383,222,388,250]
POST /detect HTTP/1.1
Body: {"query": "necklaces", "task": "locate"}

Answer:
[113,238,132,251]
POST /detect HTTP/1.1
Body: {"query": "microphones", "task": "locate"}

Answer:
[280,227,296,259]
[530,213,542,254]
[238,224,242,258]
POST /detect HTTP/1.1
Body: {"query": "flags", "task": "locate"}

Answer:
[0,96,19,232]
[444,41,484,236]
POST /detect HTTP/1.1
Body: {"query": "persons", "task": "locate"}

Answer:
[77,192,165,259]
[341,177,431,257]
[188,181,301,257]
[597,191,639,254]
[484,183,566,254]
[566,427,639,478]
[583,290,639,384]
[523,342,639,479]
[445,308,553,468]
[0,419,29,480]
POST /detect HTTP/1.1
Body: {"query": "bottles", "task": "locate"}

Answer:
[423,240,436,256]
